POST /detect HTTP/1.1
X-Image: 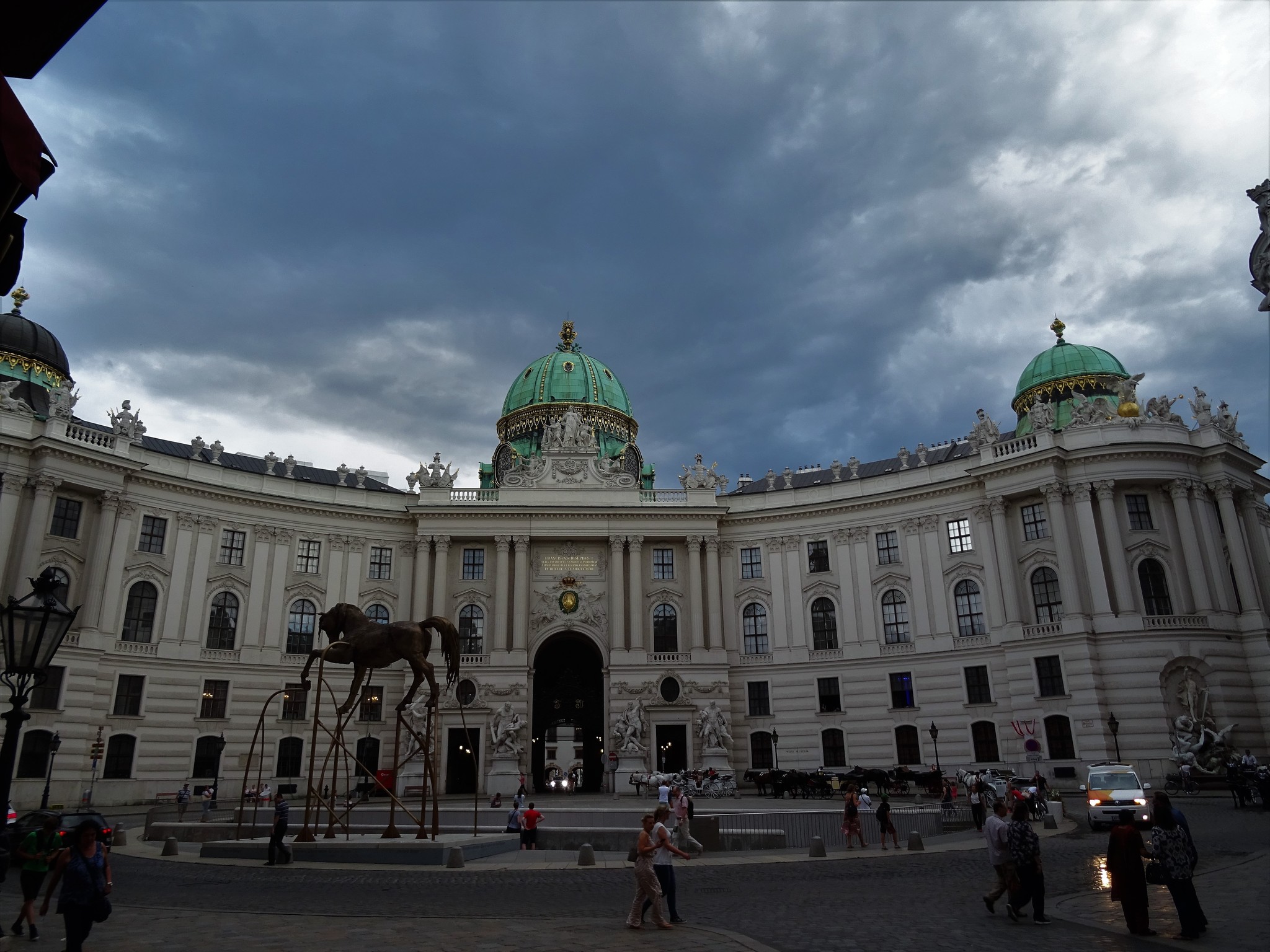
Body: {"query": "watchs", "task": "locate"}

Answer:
[106,882,113,886]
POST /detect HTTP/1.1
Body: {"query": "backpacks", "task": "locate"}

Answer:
[876,803,885,823]
[680,795,694,820]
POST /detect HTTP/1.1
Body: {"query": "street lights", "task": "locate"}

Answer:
[928,721,940,771]
[0,565,83,939]
[771,727,779,771]
[1107,711,1121,762]
[208,732,227,809]
[661,746,665,772]
[360,733,371,802]
[40,729,61,808]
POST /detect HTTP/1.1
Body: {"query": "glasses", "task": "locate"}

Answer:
[647,821,655,824]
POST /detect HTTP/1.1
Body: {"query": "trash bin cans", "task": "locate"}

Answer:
[82,790,91,803]
[52,804,64,810]
[260,790,272,807]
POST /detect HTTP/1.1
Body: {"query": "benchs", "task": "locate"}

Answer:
[154,792,179,804]
[404,786,431,797]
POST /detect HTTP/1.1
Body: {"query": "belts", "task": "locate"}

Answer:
[676,817,681,820]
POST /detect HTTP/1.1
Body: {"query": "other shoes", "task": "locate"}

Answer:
[1173,931,1193,940]
[847,843,901,850]
[942,811,958,817]
[698,845,703,856]
[976,825,982,832]
[1131,927,1157,936]
[1027,817,1039,821]
[984,895,1051,924]
[10,924,25,936]
[29,929,39,941]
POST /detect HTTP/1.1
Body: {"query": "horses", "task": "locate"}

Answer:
[854,765,890,796]
[743,769,811,800]
[630,772,680,799]
[300,602,463,714]
[1224,760,1245,807]
[956,768,987,797]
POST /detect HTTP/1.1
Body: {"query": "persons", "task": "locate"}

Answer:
[490,792,502,809]
[940,771,1050,822]
[1176,760,1195,796]
[200,784,213,823]
[490,700,528,760]
[243,783,272,803]
[520,802,545,849]
[658,780,674,810]
[264,794,291,865]
[615,700,650,751]
[876,793,901,850]
[174,781,192,823]
[5,348,1247,498]
[627,813,673,931]
[567,770,580,794]
[1240,748,1257,772]
[692,767,716,795]
[38,819,112,952]
[699,697,730,750]
[401,692,439,753]
[343,794,352,807]
[514,788,525,809]
[507,802,521,833]
[857,787,872,809]
[11,816,62,941]
[639,804,691,924]
[842,784,870,849]
[326,790,337,808]
[1105,791,1209,940]
[976,799,1053,927]
[669,786,703,857]
[517,772,529,797]
[634,770,641,795]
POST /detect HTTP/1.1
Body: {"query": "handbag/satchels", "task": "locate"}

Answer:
[1145,856,1166,885]
[92,896,112,922]
[202,795,212,801]
[1021,790,1032,799]
[847,793,857,815]
[177,796,182,804]
[628,843,638,863]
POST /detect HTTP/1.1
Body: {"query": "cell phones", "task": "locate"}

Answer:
[659,838,665,843]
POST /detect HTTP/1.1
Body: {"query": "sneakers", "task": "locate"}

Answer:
[629,917,687,929]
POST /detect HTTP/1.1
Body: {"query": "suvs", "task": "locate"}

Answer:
[6,799,114,869]
[1079,762,1153,830]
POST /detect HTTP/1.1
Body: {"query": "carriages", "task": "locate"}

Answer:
[1226,762,1270,809]
[628,771,739,799]
[956,767,1031,808]
[743,764,948,798]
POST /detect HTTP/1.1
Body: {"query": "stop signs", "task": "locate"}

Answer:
[608,753,617,761]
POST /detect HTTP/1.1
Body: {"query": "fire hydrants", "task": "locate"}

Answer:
[323,784,329,799]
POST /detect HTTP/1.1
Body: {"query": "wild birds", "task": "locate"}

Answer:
[1204,724,1239,746]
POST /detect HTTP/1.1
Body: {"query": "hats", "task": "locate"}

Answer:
[184,781,190,786]
[861,788,867,793]
[1245,749,1251,752]
[881,794,890,800]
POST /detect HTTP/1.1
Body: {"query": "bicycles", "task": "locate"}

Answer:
[567,781,576,796]
[1164,773,1200,795]
[1034,797,1046,822]
